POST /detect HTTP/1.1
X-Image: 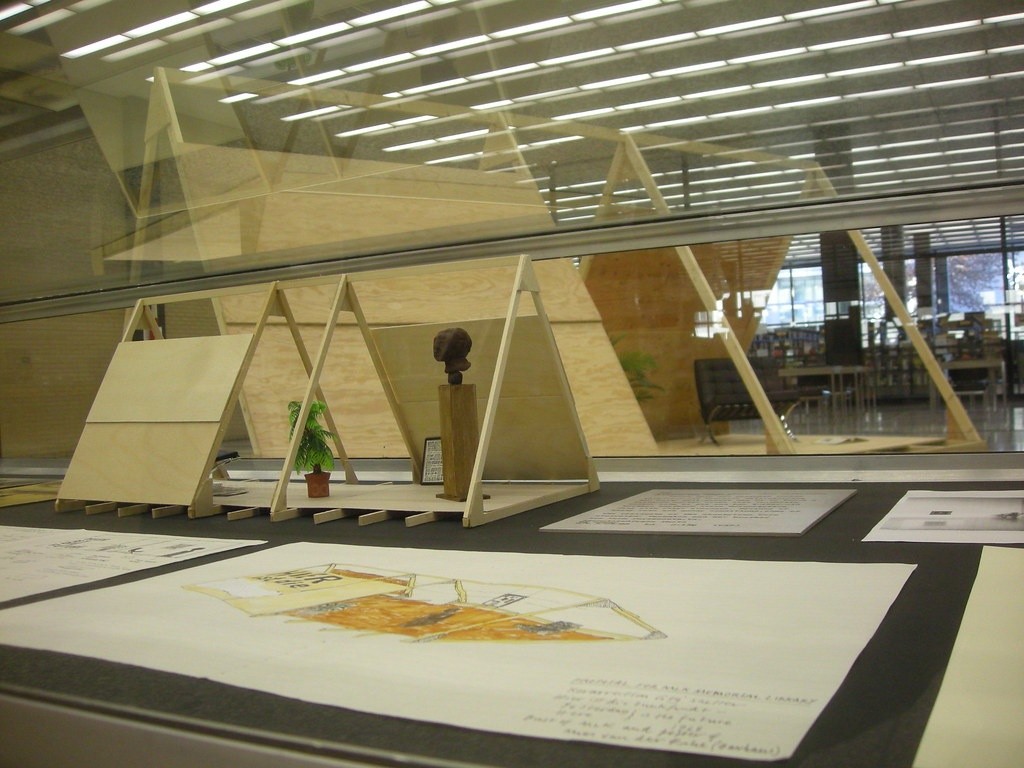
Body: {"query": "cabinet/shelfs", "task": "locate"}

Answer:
[752,314,1024,405]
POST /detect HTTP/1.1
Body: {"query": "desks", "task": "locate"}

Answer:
[779,365,869,416]
[930,359,1006,414]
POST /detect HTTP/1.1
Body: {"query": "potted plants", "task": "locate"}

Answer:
[288,400,338,498]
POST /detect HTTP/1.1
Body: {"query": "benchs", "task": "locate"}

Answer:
[693,357,801,447]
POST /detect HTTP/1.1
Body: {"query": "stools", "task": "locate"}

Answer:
[801,396,825,415]
